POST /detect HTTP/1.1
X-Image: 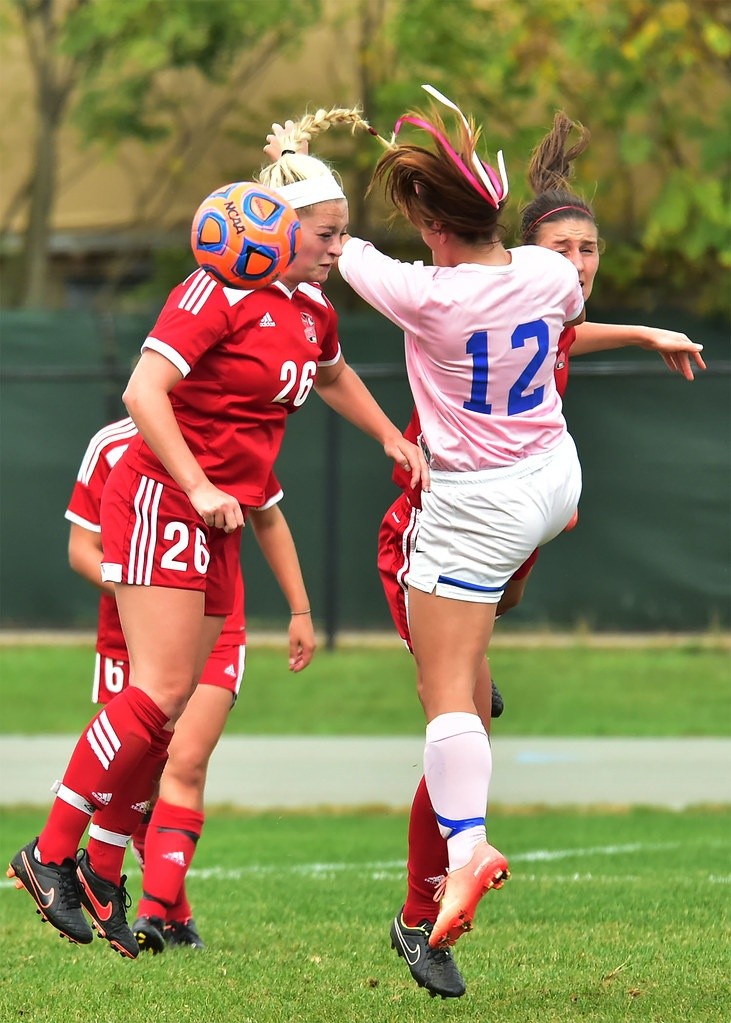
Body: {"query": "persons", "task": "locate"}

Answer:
[63,371,323,951]
[377,113,707,997]
[5,105,433,962]
[262,86,586,950]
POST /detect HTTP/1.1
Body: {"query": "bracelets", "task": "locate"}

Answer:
[290,610,311,615]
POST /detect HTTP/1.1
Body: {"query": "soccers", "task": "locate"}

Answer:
[190,180,303,291]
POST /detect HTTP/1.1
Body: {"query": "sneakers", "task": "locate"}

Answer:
[425,842,511,949]
[133,915,166,956]
[491,678,504,718]
[163,916,204,948]
[6,837,93,945]
[564,508,578,531]
[76,849,140,959]
[389,903,466,1000]
